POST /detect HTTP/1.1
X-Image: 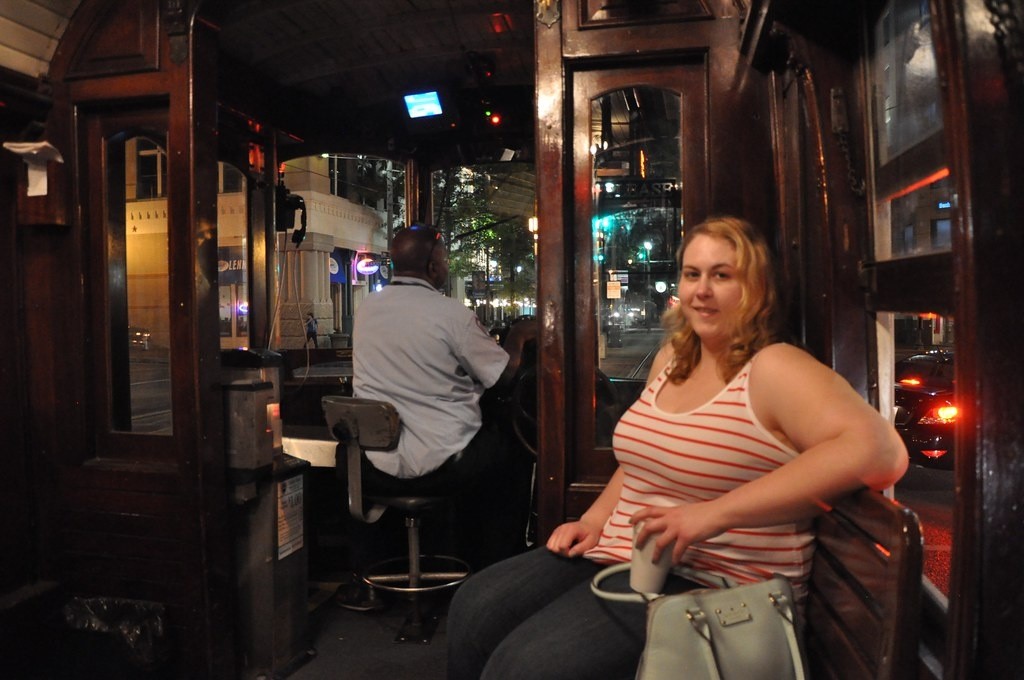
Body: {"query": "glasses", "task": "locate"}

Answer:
[415,222,442,268]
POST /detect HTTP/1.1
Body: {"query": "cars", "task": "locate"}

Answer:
[600,299,659,349]
[128,326,153,352]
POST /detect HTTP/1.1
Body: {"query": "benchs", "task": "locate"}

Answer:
[795,486,926,680]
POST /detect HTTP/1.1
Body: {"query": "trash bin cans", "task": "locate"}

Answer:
[607,325,623,348]
[328,333,350,349]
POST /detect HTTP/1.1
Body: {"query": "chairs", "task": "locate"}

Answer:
[321,395,469,641]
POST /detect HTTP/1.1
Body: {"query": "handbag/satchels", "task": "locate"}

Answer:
[590,561,805,680]
[313,321,317,332]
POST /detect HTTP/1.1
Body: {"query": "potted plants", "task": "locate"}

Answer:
[328,327,350,348]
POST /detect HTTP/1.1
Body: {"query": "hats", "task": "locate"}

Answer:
[307,313,313,317]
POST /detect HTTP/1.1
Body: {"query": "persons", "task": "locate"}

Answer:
[445,216,909,680]
[352,226,538,564]
[304,313,318,349]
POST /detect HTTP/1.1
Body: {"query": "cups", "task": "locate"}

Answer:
[630,519,676,594]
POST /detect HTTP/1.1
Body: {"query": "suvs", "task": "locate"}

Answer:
[867,349,955,467]
